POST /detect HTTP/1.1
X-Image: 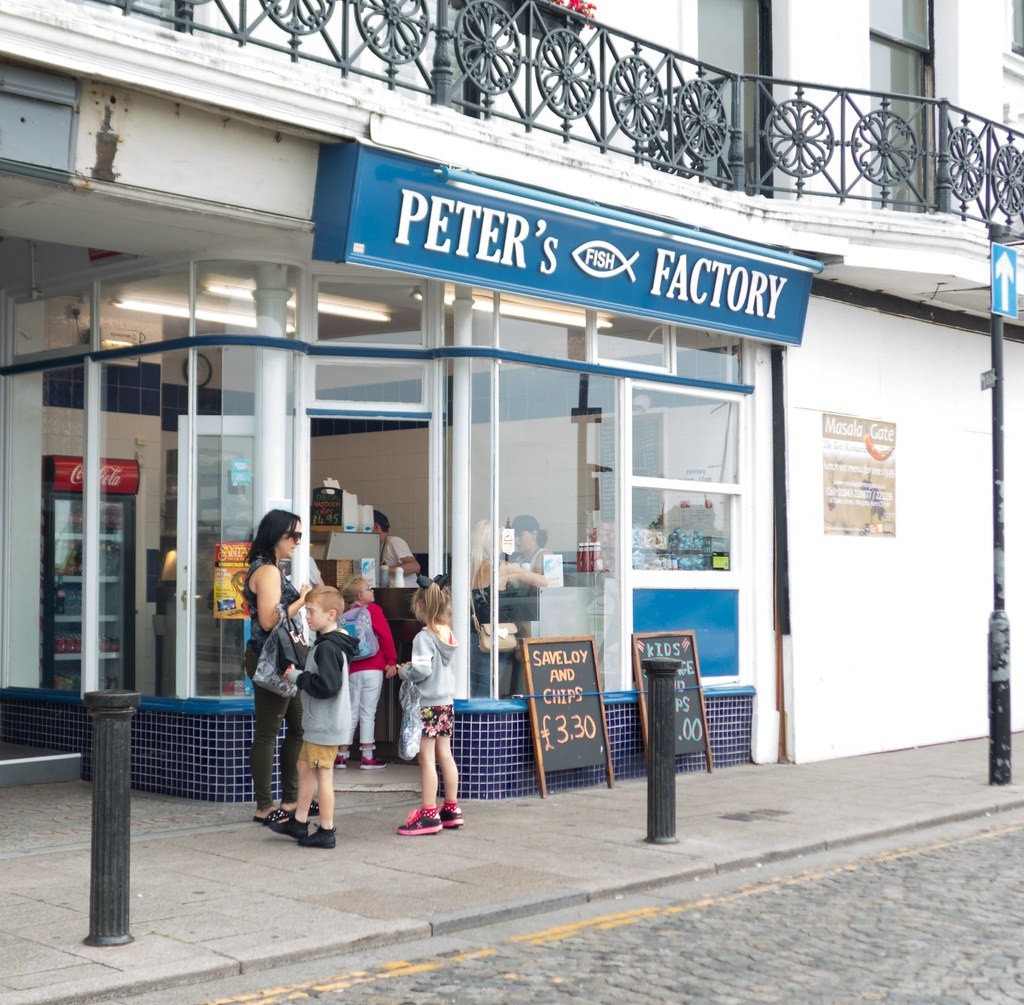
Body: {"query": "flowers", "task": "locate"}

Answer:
[548,0,597,30]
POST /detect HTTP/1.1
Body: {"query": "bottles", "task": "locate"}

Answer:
[395,564,406,588]
[577,543,602,572]
[379,561,390,588]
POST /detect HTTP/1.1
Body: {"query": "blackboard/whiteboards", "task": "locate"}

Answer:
[519,636,617,773]
[631,629,709,755]
[595,412,664,530]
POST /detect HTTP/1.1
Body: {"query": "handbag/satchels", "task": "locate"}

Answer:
[275,625,308,669]
[478,625,518,651]
[398,685,422,761]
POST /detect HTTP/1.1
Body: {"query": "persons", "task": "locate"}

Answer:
[244,509,361,849]
[373,509,421,588]
[472,514,565,698]
[334,575,398,769]
[398,572,466,835]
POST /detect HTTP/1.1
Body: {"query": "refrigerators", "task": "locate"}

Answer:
[42,452,136,696]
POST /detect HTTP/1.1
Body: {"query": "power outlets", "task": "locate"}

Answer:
[69,303,82,319]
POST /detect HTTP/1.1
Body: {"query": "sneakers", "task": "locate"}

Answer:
[272,822,307,838]
[334,752,348,768]
[397,808,441,836]
[362,755,386,768]
[442,805,462,828]
[301,829,339,848]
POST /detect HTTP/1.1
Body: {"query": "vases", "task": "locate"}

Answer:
[451,0,585,46]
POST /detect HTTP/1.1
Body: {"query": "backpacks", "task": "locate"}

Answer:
[341,607,379,660]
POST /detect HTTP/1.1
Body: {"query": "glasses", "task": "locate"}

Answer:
[362,587,372,592]
[284,530,303,537]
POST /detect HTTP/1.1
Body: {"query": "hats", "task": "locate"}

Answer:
[513,515,540,531]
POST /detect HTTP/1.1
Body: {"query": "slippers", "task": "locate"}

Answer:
[305,799,319,813]
[252,810,286,820]
